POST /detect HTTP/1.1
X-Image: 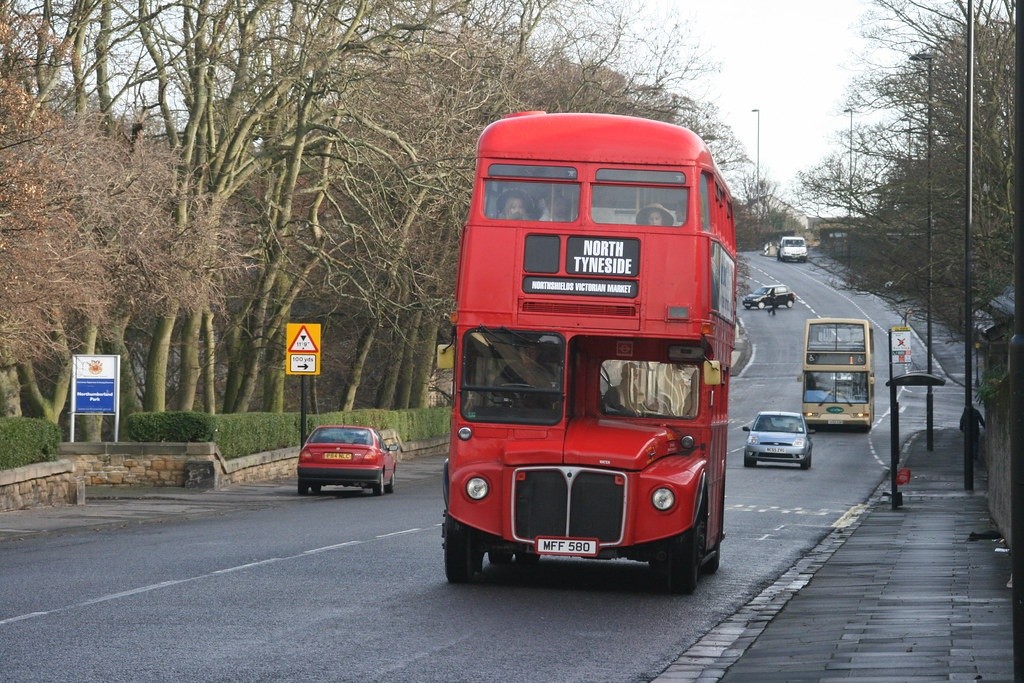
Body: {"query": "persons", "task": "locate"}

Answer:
[496,188,568,222]
[635,202,674,225]
[959,406,986,460]
[767,287,776,315]
[761,418,775,430]
[492,338,559,408]
[603,363,697,418]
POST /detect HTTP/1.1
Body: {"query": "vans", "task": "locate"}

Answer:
[776,236,810,263]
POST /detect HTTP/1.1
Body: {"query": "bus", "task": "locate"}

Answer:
[439,108,737,597]
[796,317,876,431]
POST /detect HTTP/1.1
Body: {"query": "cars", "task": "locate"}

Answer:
[743,285,795,311]
[297,426,399,496]
[740,410,813,472]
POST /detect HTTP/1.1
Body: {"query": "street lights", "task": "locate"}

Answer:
[843,108,853,272]
[908,52,935,454]
[751,109,760,248]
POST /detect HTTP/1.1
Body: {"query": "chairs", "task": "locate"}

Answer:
[355,439,367,444]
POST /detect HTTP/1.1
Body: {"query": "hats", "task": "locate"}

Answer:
[496,187,535,213]
[635,203,674,226]
